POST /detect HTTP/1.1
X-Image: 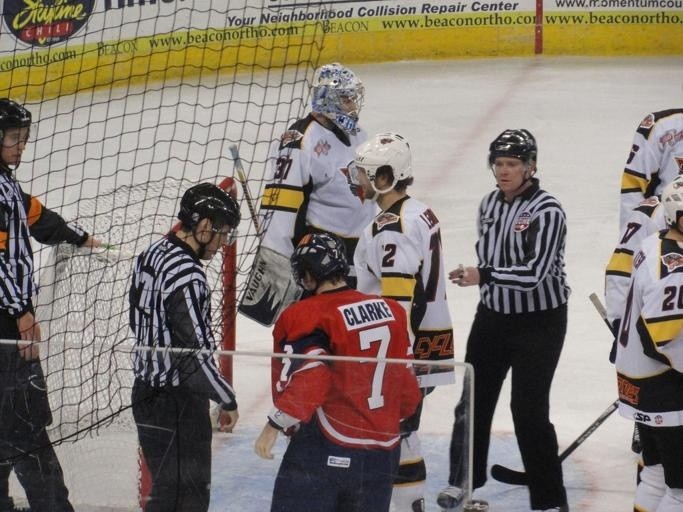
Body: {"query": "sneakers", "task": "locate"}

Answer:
[436,488,464,507]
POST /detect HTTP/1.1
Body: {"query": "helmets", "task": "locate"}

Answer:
[355,133,413,195]
[179,183,241,232]
[663,177,683,226]
[306,64,365,137]
[290,231,349,283]
[0,98,32,128]
[489,129,535,161]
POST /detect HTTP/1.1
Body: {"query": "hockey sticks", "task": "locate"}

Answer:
[491,397,621,484]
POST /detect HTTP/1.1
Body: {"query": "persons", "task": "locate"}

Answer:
[617,108,682,240]
[0,95,118,511]
[346,132,453,443]
[435,127,574,512]
[250,230,424,512]
[614,172,682,511]
[255,63,372,317]
[599,188,665,486]
[129,182,240,512]
